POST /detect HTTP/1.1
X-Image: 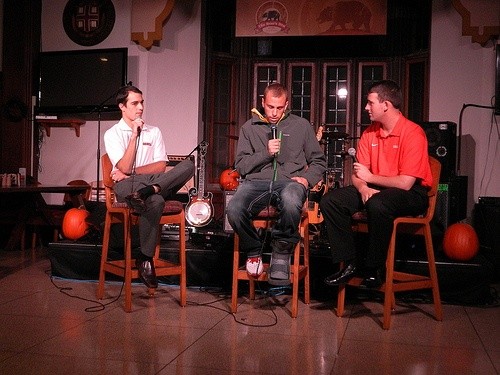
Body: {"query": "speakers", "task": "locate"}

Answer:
[165,155,196,202]
[420,120,469,243]
[223,191,236,233]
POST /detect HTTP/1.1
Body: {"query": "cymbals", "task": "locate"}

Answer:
[225,135,239,140]
[321,131,350,139]
[332,151,352,157]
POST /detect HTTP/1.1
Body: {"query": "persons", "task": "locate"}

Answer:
[228,83,328,285]
[104,87,195,288]
[320,80,433,290]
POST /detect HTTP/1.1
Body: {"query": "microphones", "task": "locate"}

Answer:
[137,126,142,137]
[348,148,358,163]
[270,125,278,158]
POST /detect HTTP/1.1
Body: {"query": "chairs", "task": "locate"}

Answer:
[25,180,91,249]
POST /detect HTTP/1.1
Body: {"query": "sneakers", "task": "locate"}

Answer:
[246,255,264,277]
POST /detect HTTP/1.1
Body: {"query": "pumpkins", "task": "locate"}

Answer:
[219,168,241,191]
[62,205,92,240]
[442,220,479,260]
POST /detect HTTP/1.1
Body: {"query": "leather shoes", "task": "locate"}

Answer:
[326,262,367,285]
[135,255,158,289]
[360,271,385,289]
[126,194,146,213]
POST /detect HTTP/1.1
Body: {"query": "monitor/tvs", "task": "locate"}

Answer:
[36,47,129,113]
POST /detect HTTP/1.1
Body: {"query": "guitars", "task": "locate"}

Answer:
[304,125,328,224]
[185,141,214,227]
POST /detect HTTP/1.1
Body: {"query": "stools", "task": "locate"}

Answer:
[98,154,185,313]
[336,156,443,329]
[231,197,310,318]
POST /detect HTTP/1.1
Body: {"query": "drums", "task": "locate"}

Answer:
[316,171,335,189]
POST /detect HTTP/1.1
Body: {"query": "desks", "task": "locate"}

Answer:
[0,183,92,250]
